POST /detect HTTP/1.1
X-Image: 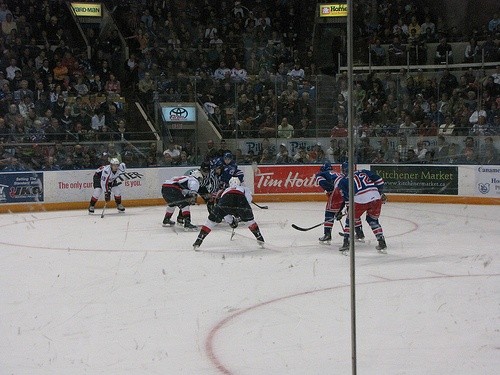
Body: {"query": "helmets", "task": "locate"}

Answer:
[225,153,235,160]
[110,158,120,165]
[229,177,241,186]
[320,160,334,171]
[202,161,211,172]
[190,170,203,180]
[341,163,348,174]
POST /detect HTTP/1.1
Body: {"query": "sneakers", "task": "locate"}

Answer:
[177,215,184,226]
[87,205,95,215]
[339,238,350,255]
[319,230,332,244]
[184,221,198,232]
[192,229,210,250]
[162,217,176,226]
[355,225,366,244]
[376,238,388,254]
[254,231,266,248]
[117,203,125,214]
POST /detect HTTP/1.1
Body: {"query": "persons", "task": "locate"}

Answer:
[193,177,265,251]
[337,162,388,254]
[88,158,125,214]
[210,152,245,188]
[0,0,500,172]
[315,160,365,246]
[161,170,203,232]
[176,161,218,226]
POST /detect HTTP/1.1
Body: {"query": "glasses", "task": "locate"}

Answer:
[281,146,284,148]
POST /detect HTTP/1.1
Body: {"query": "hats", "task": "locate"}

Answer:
[280,143,285,146]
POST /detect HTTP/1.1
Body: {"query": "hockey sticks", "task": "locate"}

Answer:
[230,228,236,241]
[291,213,348,232]
[251,201,269,210]
[339,218,345,237]
[100,201,108,218]
